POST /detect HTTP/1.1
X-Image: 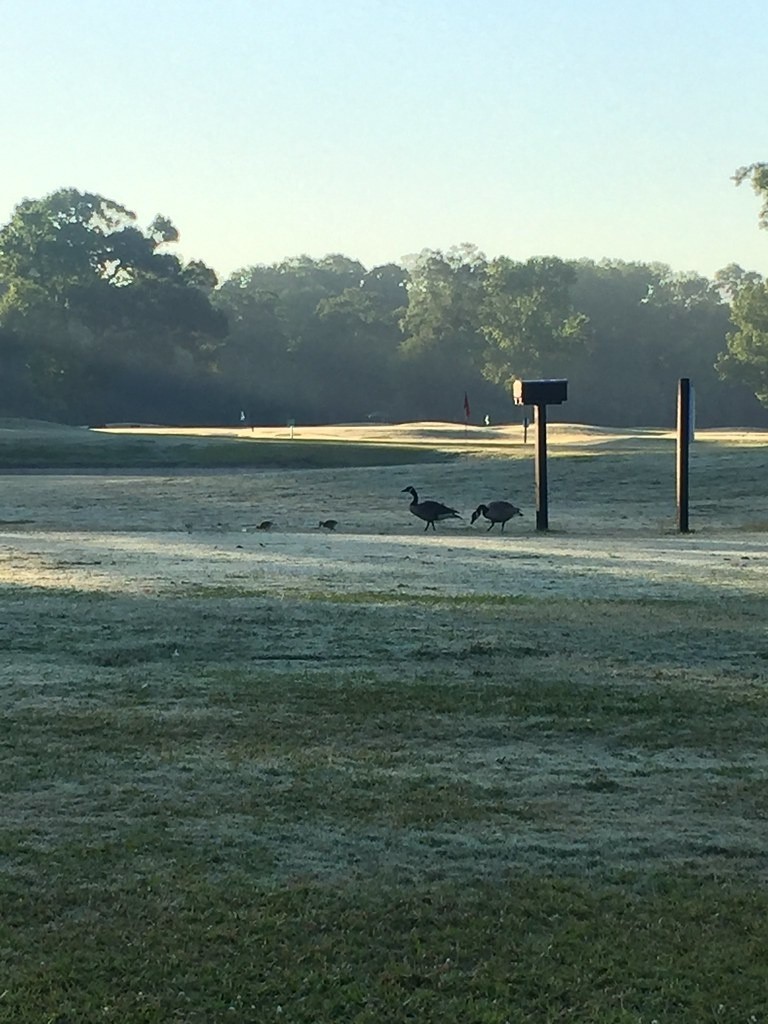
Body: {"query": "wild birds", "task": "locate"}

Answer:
[401,486,463,532]
[256,521,273,533]
[470,501,524,532]
[318,520,338,532]
